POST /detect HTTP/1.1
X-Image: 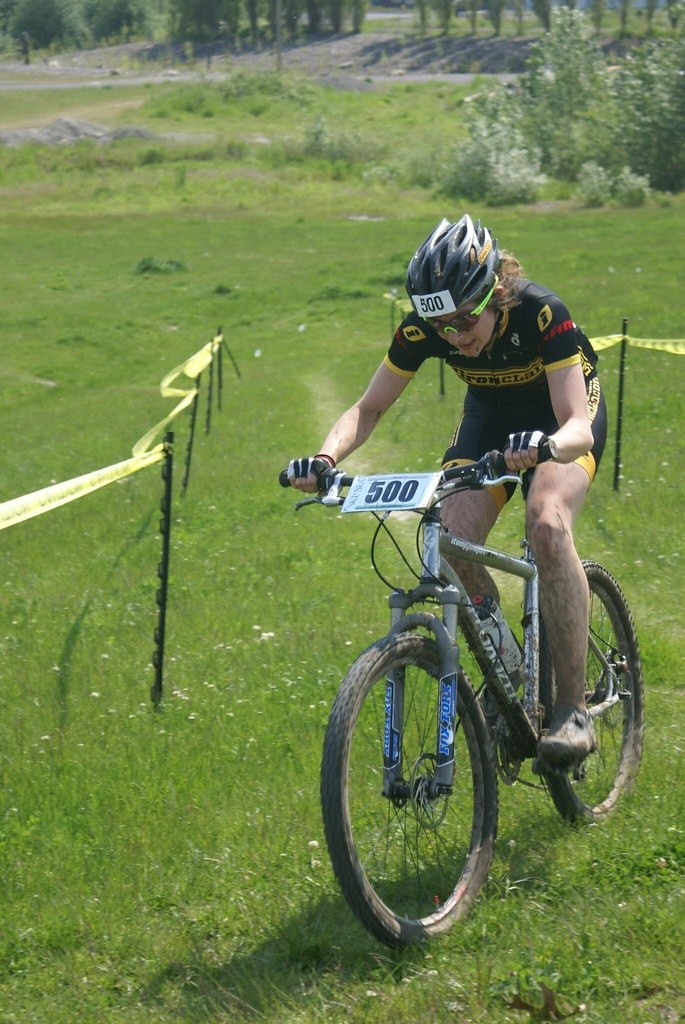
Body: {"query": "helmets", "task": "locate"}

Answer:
[405,214,500,318]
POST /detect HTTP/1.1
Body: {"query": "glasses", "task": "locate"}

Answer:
[422,273,499,334]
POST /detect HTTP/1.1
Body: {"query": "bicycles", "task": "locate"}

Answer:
[276,438,645,954]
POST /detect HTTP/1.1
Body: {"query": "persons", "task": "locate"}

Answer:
[286,213,607,767]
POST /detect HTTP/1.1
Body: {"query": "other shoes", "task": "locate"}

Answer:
[478,669,523,736]
[536,704,599,765]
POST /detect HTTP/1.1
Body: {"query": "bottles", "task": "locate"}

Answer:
[471,596,521,675]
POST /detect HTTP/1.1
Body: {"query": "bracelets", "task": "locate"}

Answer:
[314,454,336,467]
